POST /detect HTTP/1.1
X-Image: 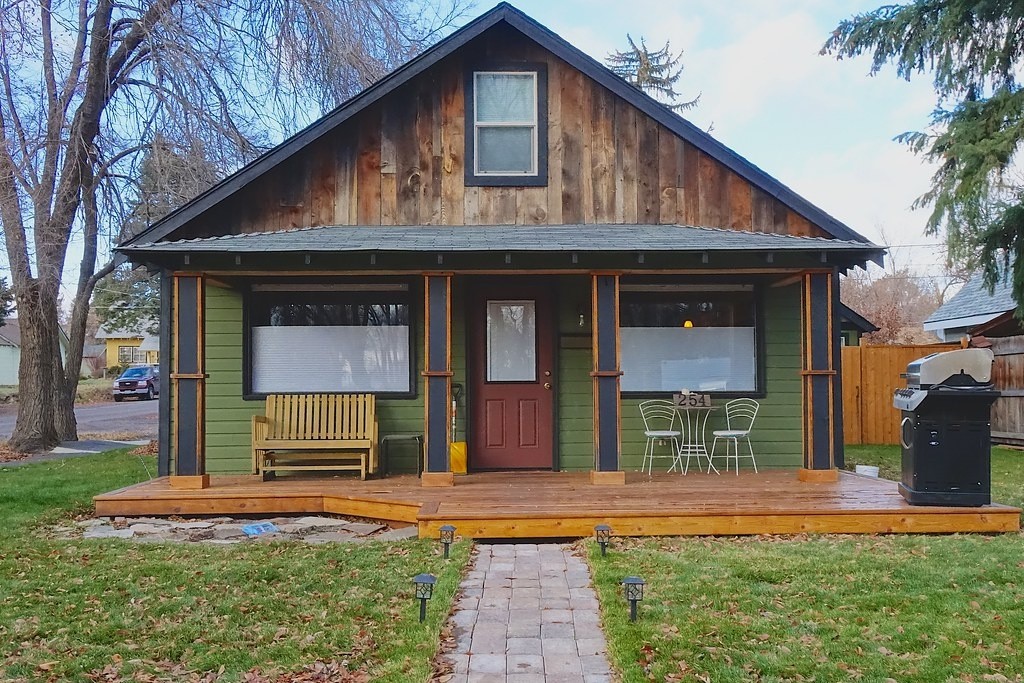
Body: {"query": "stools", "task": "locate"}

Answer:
[381,435,422,478]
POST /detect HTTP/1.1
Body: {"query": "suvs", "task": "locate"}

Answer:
[112,366,160,402]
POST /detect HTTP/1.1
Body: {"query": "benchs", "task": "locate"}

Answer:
[250,395,380,479]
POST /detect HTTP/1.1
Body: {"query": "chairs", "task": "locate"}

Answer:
[638,399,684,476]
[707,398,759,475]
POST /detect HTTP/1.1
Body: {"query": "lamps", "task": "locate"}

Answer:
[623,577,643,600]
[595,525,610,543]
[414,572,436,600]
[438,525,455,544]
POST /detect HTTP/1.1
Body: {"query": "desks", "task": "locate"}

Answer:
[668,405,722,475]
